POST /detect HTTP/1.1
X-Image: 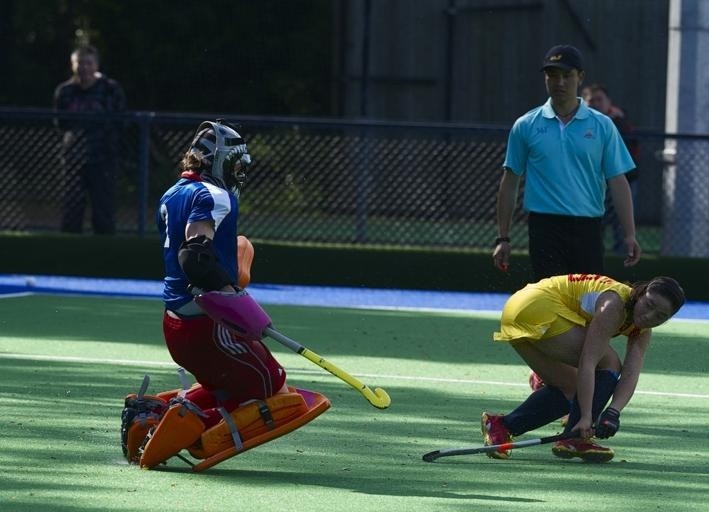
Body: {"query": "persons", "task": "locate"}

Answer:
[480,273,684,461]
[52,47,130,234]
[120,119,331,471]
[493,43,642,425]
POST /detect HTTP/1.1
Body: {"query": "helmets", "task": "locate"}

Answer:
[192,119,251,202]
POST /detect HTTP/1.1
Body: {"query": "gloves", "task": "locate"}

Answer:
[594,407,620,439]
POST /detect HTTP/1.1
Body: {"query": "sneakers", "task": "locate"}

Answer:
[552,436,614,462]
[121,407,160,465]
[480,410,512,460]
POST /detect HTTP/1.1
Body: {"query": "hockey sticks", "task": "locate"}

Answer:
[423,428,596,462]
[186,284,391,409]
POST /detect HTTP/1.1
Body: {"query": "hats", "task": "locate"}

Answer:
[542,43,588,72]
